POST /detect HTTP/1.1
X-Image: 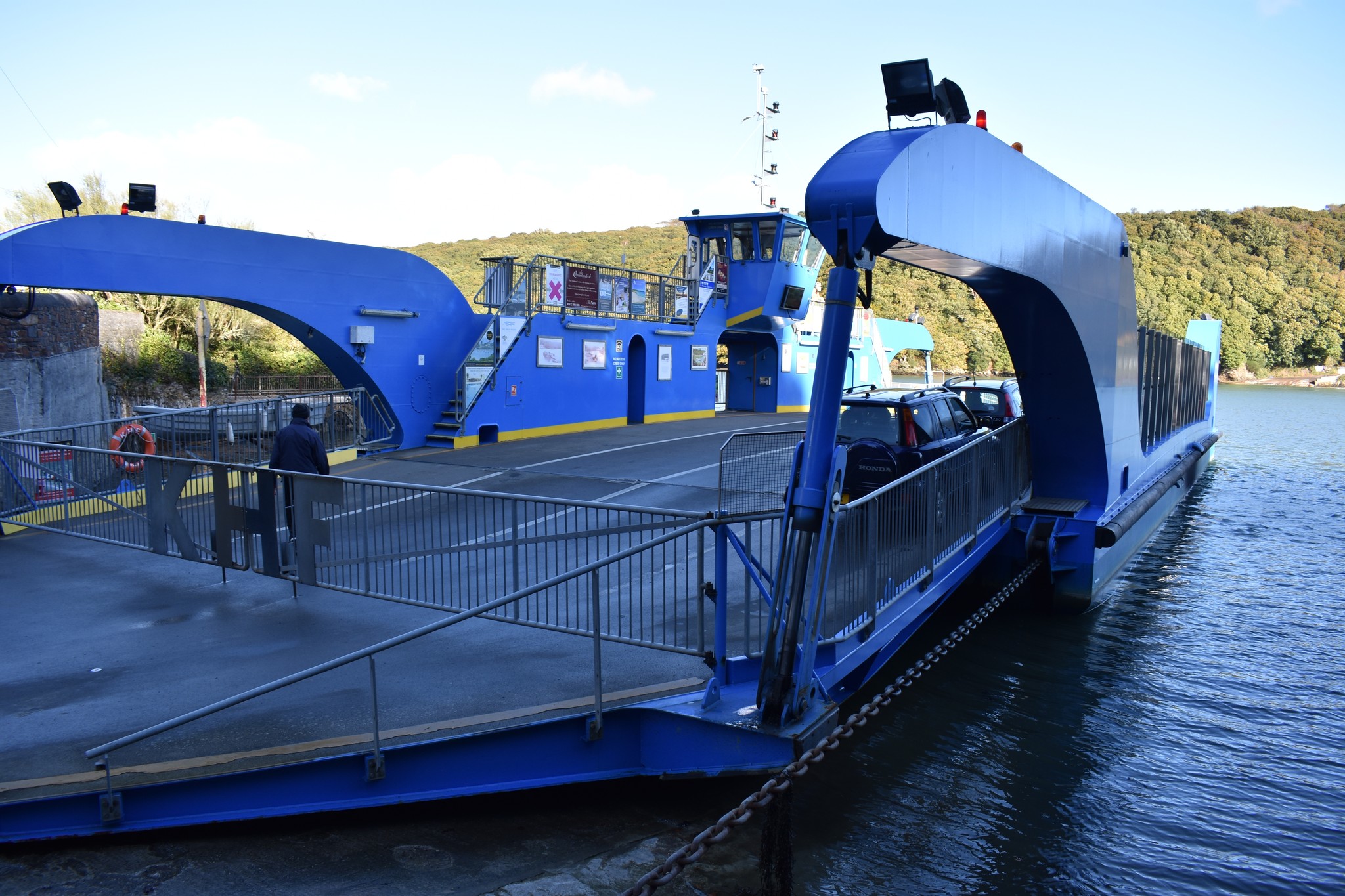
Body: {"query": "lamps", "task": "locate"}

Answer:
[413,311,420,318]
[565,323,616,331]
[655,329,694,337]
[307,329,314,339]
[128,183,157,212]
[360,308,414,318]
[879,58,938,129]
[692,209,700,217]
[47,181,83,217]
[6,285,16,295]
[936,77,971,125]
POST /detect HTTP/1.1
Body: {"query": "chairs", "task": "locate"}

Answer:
[837,410,866,442]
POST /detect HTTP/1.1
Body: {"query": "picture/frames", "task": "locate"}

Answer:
[657,344,672,381]
[536,335,564,368]
[781,343,792,372]
[582,339,606,369]
[691,344,708,370]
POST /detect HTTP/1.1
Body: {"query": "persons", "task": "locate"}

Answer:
[267,403,330,557]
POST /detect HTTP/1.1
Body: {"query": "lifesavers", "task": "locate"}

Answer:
[109,423,156,474]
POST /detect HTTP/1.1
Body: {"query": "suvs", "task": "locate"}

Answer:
[782,384,995,545]
[941,375,1026,459]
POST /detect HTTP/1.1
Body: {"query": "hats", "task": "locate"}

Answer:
[292,404,310,419]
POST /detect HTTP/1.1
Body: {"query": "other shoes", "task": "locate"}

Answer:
[289,535,296,542]
